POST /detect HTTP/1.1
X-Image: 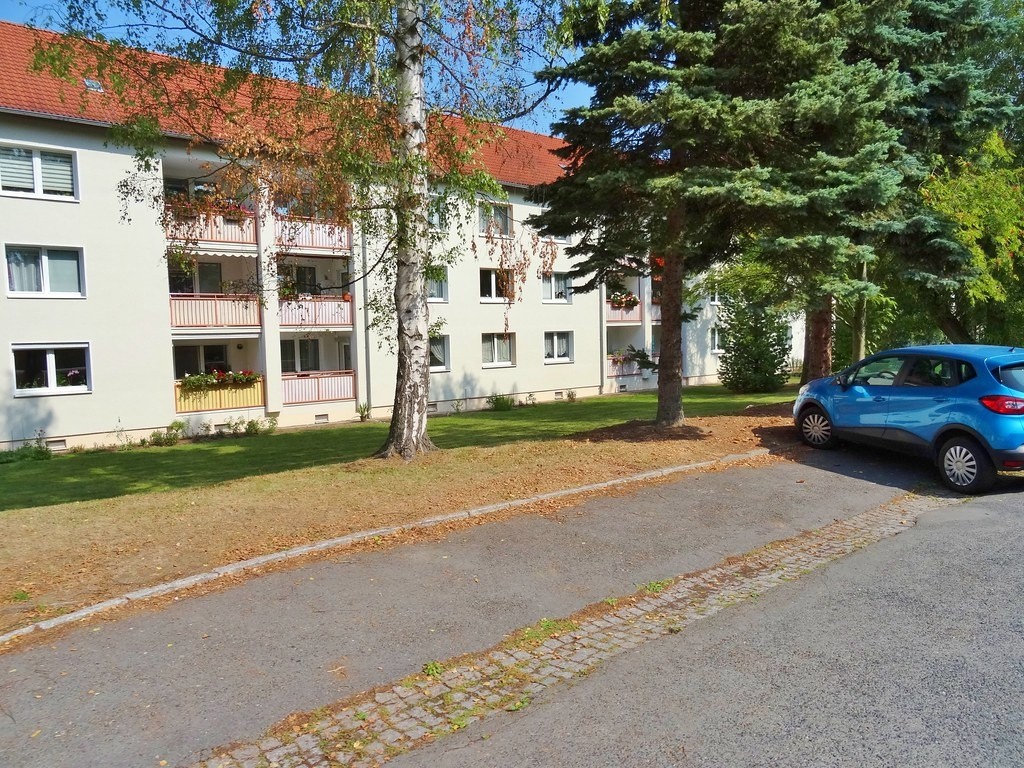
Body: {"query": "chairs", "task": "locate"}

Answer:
[907,359,941,386]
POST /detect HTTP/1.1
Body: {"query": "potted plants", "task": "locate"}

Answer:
[356,403,372,421]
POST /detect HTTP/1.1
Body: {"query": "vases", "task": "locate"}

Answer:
[179,208,197,217]
[612,301,635,308]
[223,214,241,220]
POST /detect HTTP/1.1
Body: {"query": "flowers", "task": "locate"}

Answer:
[225,205,246,215]
[180,368,262,390]
[177,201,197,208]
[610,292,640,307]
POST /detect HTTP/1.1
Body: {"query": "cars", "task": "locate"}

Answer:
[792,345,1024,493]
